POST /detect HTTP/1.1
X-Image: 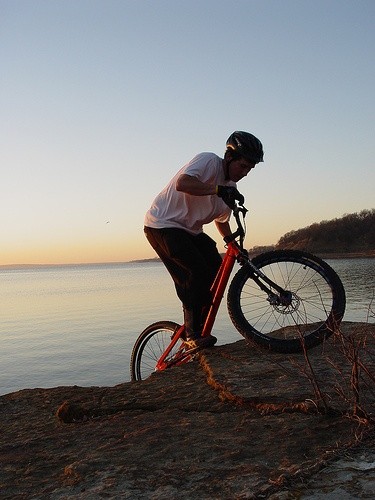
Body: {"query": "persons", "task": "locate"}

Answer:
[143,131,263,353]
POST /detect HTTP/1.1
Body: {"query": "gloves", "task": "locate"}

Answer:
[216,185,244,210]
[236,248,248,266]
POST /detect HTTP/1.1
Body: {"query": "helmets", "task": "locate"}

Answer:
[224,131,264,182]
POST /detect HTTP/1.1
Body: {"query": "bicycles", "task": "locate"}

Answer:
[130,190,346,381]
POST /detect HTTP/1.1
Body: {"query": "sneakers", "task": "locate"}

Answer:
[184,334,217,353]
